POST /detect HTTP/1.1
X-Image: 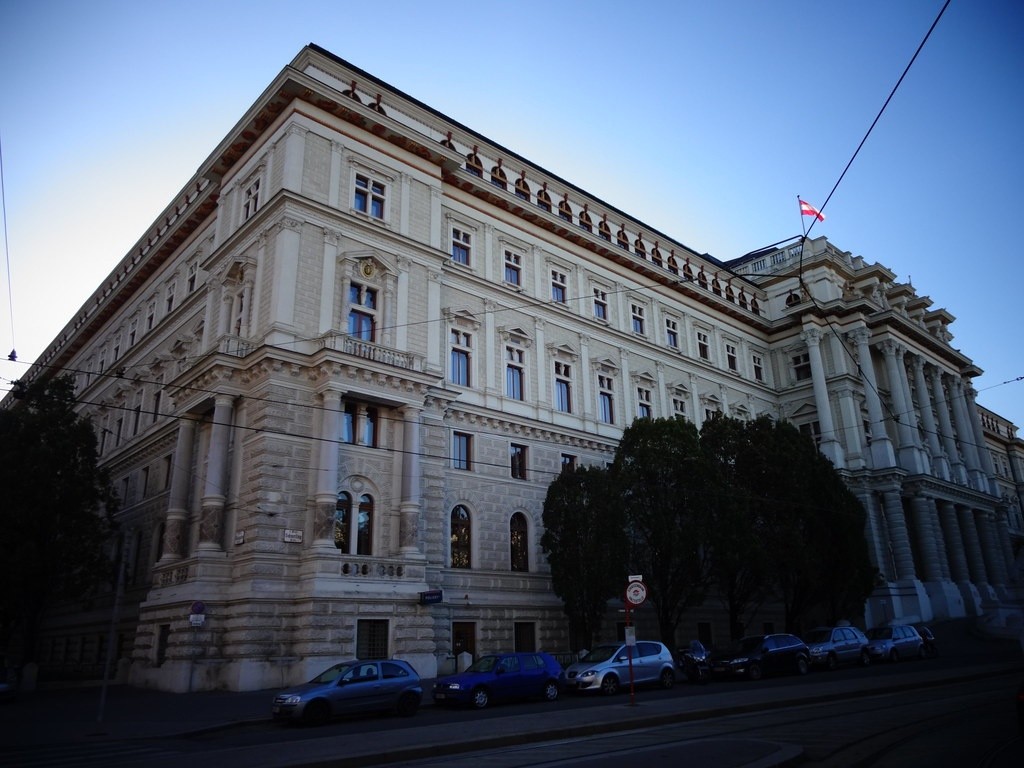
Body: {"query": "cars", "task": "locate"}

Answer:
[563,640,678,696]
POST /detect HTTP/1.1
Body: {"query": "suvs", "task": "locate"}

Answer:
[804,625,871,674]
[710,632,813,682]
[863,623,927,662]
[275,659,424,728]
[428,652,565,711]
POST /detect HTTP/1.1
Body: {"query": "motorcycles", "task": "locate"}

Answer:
[677,640,711,685]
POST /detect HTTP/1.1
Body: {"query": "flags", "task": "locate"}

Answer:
[799,200,826,223]
